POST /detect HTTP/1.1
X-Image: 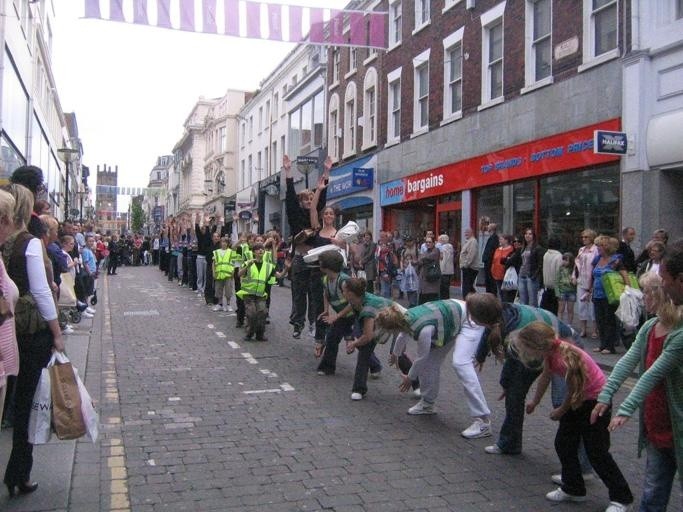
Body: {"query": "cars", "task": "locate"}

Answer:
[516,218,567,246]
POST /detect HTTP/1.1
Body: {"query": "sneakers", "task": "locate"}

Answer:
[351,392,362,399]
[604,501,634,512]
[414,388,420,396]
[485,446,503,454]
[79,301,96,318]
[294,323,304,337]
[546,488,585,502]
[552,473,594,485]
[408,399,435,414]
[206,302,233,312]
[462,418,491,438]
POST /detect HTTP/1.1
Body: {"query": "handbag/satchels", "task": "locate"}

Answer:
[423,260,441,280]
[15,291,60,333]
[59,272,76,307]
[27,352,98,445]
[294,228,316,245]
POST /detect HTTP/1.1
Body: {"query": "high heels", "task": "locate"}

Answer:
[3,479,38,495]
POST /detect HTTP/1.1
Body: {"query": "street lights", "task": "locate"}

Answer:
[295,160,316,190]
[57,147,81,222]
[75,190,91,226]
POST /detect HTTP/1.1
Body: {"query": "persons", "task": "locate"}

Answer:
[1,165,119,499]
[281,151,333,339]
[338,275,423,401]
[289,204,357,358]
[346,224,455,309]
[657,238,682,307]
[119,209,283,343]
[589,269,682,511]
[458,222,680,356]
[464,291,596,486]
[505,320,634,511]
[313,249,384,381]
[377,294,495,440]
